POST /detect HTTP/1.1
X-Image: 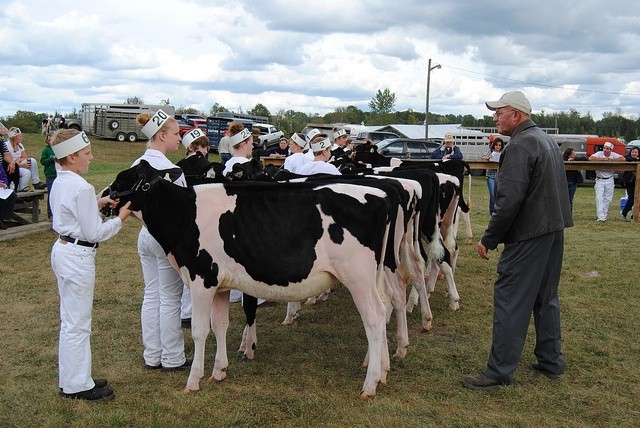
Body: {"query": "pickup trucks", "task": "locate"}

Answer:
[252,123,285,150]
[176,120,206,144]
[53,117,82,131]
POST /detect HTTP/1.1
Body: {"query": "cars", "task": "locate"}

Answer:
[373,137,446,161]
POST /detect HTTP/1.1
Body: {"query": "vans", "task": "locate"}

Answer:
[356,132,400,143]
[183,115,204,120]
[206,116,256,154]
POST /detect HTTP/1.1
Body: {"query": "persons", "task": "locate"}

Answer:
[481,138,505,215]
[270,137,291,157]
[461,91,574,390]
[620,147,640,222]
[343,139,354,152]
[40,131,59,220]
[222,124,272,307]
[129,109,193,369]
[431,134,462,164]
[332,130,348,157]
[589,142,626,223]
[6,126,48,192]
[303,128,322,161]
[45,115,54,130]
[489,134,495,144]
[284,133,311,174]
[58,117,68,130]
[48,129,131,401]
[0,121,21,215]
[218,134,233,165]
[181,127,212,325]
[296,133,341,176]
[1,166,9,227]
[562,149,583,214]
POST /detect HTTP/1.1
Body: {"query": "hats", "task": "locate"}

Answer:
[485,91,532,115]
[445,134,454,142]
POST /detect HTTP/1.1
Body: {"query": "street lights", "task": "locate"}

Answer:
[423,57,442,137]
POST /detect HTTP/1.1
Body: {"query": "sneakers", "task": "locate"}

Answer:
[181,318,191,328]
[3,220,15,226]
[620,210,627,221]
[58,379,107,396]
[33,181,47,189]
[258,301,276,307]
[163,358,194,369]
[18,186,31,192]
[49,216,53,221]
[0,220,7,229]
[145,363,162,369]
[62,387,114,402]
[597,218,607,221]
[461,372,512,390]
[528,364,564,378]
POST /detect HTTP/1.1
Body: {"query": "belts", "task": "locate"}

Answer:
[597,174,614,179]
[60,235,98,247]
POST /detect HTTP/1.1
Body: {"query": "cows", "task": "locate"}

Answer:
[345,141,474,245]
[226,156,461,326]
[303,147,462,306]
[174,150,423,364]
[95,158,394,402]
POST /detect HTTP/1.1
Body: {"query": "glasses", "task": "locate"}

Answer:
[494,144,501,146]
[493,109,518,118]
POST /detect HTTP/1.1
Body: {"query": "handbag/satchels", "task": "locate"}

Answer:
[575,171,583,183]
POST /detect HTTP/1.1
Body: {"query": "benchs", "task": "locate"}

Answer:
[15,188,44,225]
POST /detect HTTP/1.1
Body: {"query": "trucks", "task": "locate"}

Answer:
[447,124,639,187]
[92,104,185,141]
[298,122,372,145]
